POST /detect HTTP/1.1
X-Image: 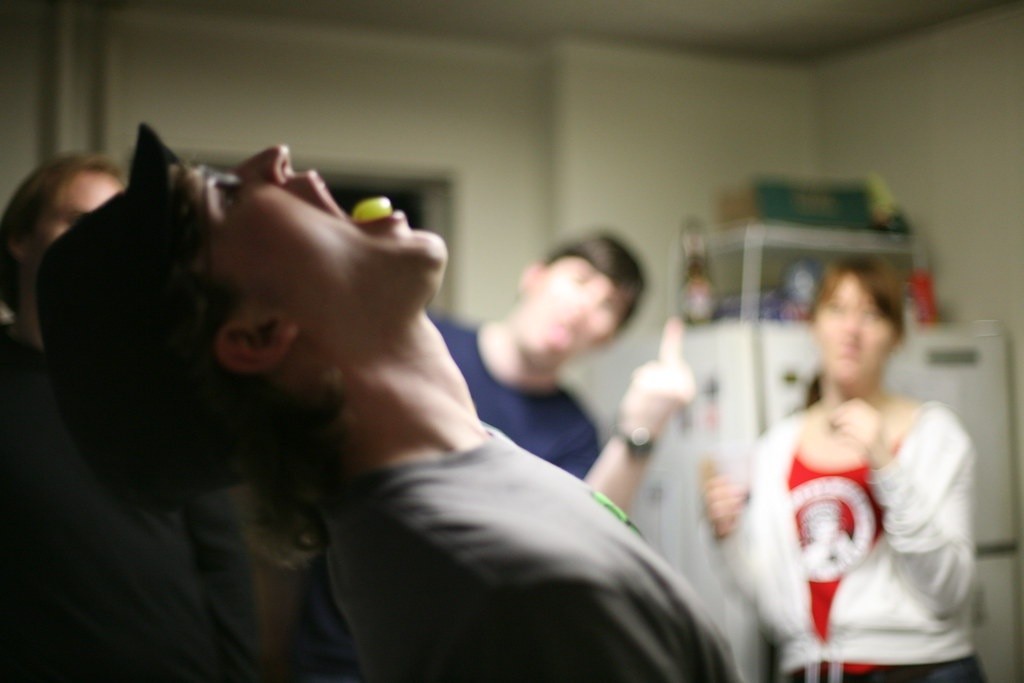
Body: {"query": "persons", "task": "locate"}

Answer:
[0,122,743,683]
[700,251,988,683]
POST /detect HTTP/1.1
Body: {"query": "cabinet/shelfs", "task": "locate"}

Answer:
[660,221,1024,683]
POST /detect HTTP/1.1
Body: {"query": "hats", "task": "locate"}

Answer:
[33,125,240,513]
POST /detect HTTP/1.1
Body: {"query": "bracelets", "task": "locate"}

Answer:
[616,429,655,457]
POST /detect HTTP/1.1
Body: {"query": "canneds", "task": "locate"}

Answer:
[909,268,937,322]
